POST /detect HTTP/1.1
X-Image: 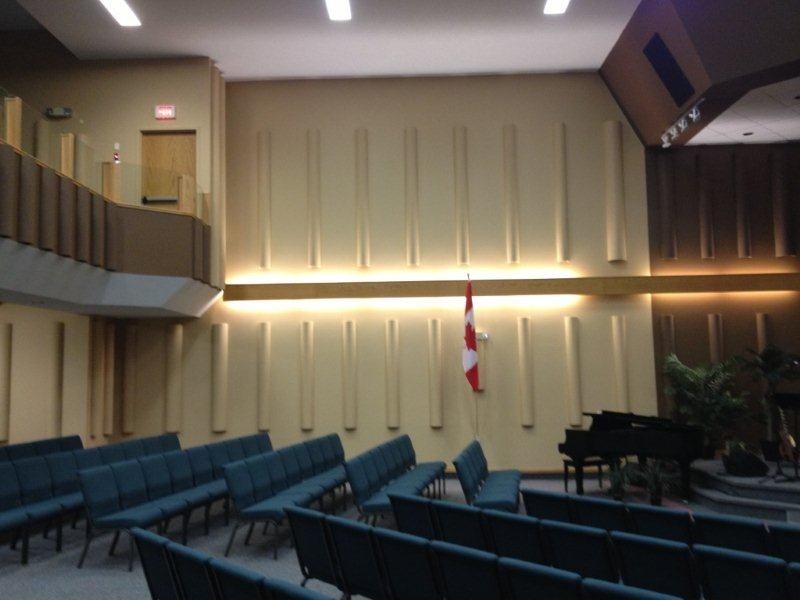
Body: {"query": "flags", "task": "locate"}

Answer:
[461,279,480,393]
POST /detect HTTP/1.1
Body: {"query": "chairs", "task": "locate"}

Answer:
[0,433,800,600]
